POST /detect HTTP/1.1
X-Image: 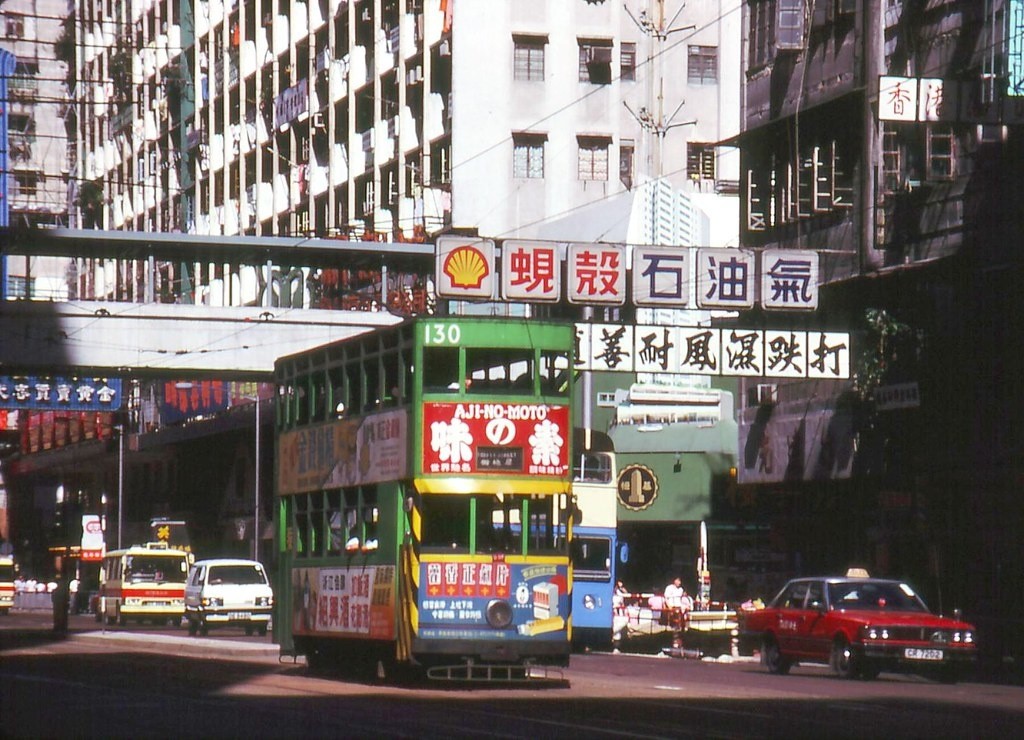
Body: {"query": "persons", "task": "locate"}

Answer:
[612,577,694,612]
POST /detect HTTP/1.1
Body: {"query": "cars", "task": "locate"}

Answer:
[183,555,273,639]
[737,571,985,683]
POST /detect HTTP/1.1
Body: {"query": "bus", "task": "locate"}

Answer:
[270,309,590,693]
[0,553,15,612]
[485,422,631,652]
[97,547,194,627]
